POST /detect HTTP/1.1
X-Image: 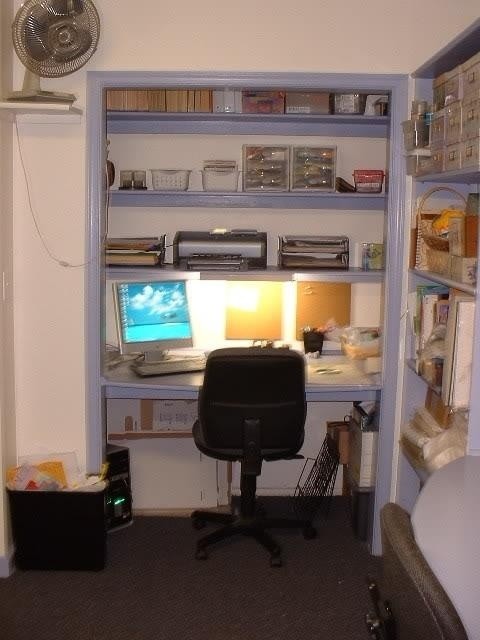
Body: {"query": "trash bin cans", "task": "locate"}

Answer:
[5,472,113,572]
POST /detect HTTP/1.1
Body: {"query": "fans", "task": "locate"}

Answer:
[6,0,101,106]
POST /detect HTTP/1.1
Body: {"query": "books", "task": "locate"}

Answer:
[105,236,162,268]
[280,235,348,270]
[406,282,475,411]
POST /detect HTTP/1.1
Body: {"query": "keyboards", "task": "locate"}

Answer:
[130,359,206,377]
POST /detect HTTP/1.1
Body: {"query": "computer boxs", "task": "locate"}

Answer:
[106,443,134,534]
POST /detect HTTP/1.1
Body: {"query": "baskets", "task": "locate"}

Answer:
[416,187,468,251]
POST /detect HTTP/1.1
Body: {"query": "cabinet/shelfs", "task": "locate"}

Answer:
[83,64,410,559]
[394,18,479,556]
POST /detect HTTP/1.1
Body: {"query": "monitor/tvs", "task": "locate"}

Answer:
[113,279,198,362]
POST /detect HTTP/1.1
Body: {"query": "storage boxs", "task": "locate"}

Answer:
[425,47,479,171]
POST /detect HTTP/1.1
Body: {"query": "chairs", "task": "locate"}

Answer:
[191,347,318,567]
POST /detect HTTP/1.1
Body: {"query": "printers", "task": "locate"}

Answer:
[173,229,267,272]
